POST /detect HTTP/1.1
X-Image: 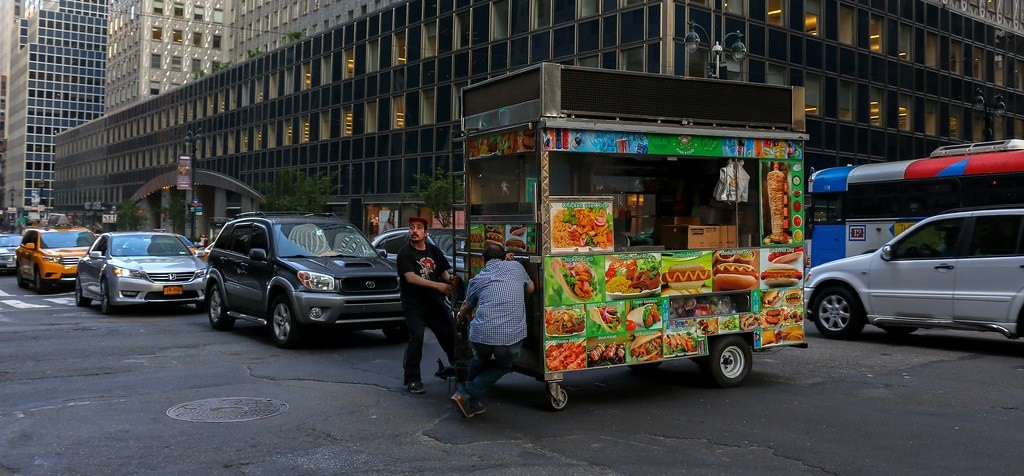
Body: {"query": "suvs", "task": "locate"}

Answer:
[800,208,1024,341]
[203,209,412,350]
[13,226,98,295]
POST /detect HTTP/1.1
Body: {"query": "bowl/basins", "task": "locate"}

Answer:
[717,250,735,264]
[738,249,755,264]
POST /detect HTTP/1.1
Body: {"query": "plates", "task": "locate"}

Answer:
[606,284,661,300]
[791,163,803,243]
[545,323,586,337]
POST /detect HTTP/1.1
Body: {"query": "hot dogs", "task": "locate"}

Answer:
[713,262,759,290]
[760,246,803,287]
[661,263,711,289]
[469,225,527,253]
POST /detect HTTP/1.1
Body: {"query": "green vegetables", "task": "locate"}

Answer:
[663,331,698,355]
[616,254,661,279]
[563,204,614,248]
[719,315,739,331]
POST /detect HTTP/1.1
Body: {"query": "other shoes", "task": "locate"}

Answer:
[451,394,469,418]
[405,380,424,393]
[468,402,487,417]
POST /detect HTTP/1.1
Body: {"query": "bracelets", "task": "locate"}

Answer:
[458,311,461,320]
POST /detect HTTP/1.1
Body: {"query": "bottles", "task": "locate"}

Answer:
[753,293,758,312]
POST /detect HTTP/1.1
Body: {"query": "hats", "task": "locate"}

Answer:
[408,217,429,226]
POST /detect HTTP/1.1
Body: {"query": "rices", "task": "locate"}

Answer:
[551,209,576,248]
[605,274,641,294]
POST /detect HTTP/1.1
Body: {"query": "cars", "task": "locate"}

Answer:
[372,225,466,287]
[73,230,209,314]
[0,234,22,273]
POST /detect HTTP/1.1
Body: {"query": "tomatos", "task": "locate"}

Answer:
[626,320,635,331]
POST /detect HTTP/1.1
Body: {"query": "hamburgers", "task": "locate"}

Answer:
[762,288,802,307]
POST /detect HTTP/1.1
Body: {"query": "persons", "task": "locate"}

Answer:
[451,244,534,418]
[919,226,961,257]
[397,217,459,393]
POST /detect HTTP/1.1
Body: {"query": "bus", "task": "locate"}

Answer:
[807,137,1024,270]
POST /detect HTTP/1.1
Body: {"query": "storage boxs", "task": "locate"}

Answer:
[663,224,737,249]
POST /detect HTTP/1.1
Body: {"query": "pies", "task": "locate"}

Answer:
[627,303,661,329]
[550,257,598,302]
[589,305,622,333]
[629,331,662,361]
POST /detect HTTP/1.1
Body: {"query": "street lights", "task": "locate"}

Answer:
[184,130,203,244]
[37,181,45,219]
[9,188,16,234]
